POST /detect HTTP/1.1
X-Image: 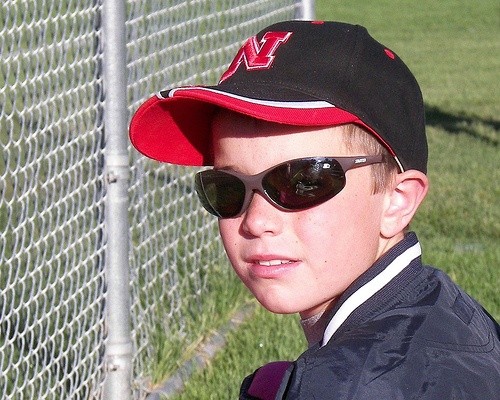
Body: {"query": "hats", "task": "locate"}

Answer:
[128,20,429,176]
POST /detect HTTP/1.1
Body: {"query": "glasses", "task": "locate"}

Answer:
[193,154,396,220]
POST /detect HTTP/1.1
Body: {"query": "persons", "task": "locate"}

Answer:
[129,21,499,400]
